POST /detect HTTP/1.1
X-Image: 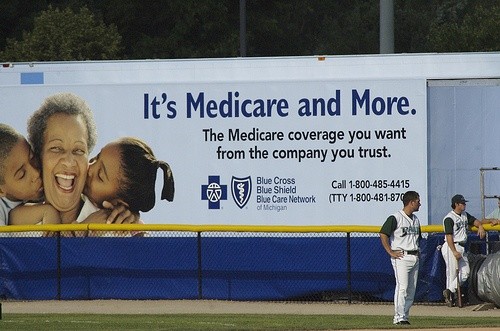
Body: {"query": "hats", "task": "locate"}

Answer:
[452,194,469,202]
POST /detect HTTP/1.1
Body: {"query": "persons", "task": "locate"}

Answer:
[0,123,140,238]
[481,196,500,227]
[8,136,174,238]
[2,91,132,237]
[441,195,485,307]
[379,191,423,325]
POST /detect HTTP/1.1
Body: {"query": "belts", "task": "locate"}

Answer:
[403,250,419,255]
[453,242,465,247]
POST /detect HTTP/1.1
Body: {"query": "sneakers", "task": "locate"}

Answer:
[453,298,458,306]
[400,321,410,325]
[443,289,454,307]
[396,322,400,325]
